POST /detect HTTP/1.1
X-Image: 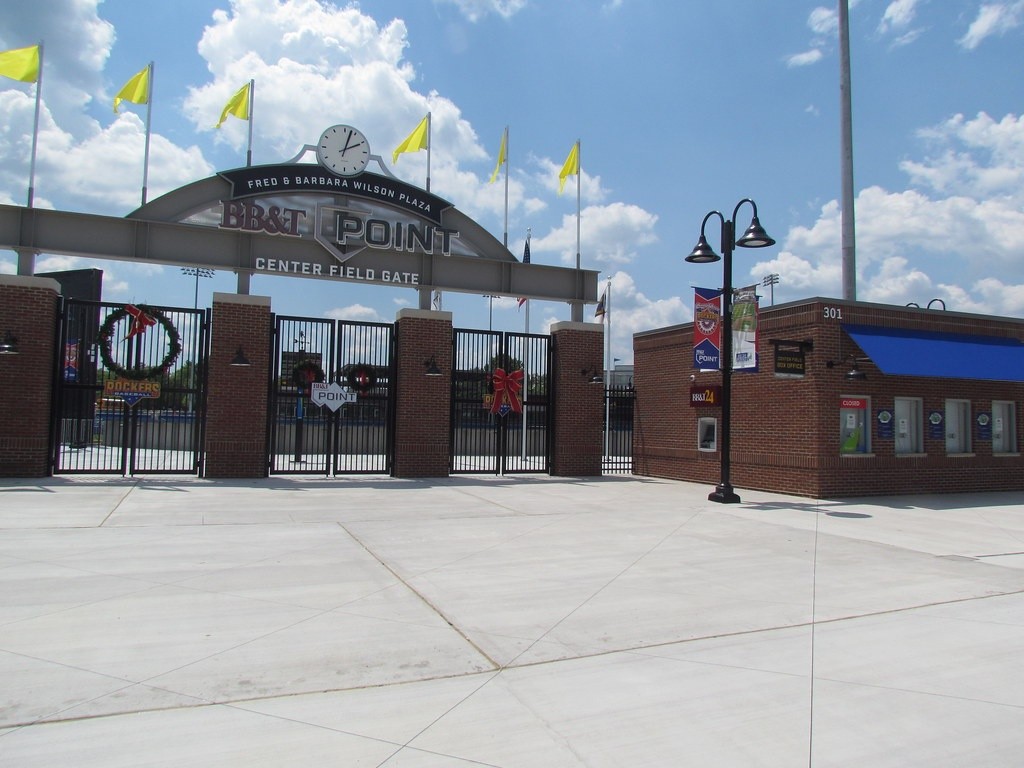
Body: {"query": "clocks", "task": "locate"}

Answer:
[316,124,370,178]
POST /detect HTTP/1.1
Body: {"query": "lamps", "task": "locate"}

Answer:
[826,354,867,381]
[230,345,250,366]
[0,329,19,354]
[425,354,442,376]
[582,365,603,384]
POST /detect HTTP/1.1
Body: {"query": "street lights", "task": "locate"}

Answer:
[684,197,778,504]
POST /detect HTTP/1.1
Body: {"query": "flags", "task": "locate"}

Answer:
[488,127,506,185]
[215,83,250,127]
[113,65,148,114]
[516,238,531,309]
[594,289,606,317]
[692,287,721,372]
[0,45,39,83]
[558,142,578,196]
[392,115,427,164]
[731,285,760,374]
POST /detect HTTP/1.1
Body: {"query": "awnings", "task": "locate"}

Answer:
[842,325,1024,383]
